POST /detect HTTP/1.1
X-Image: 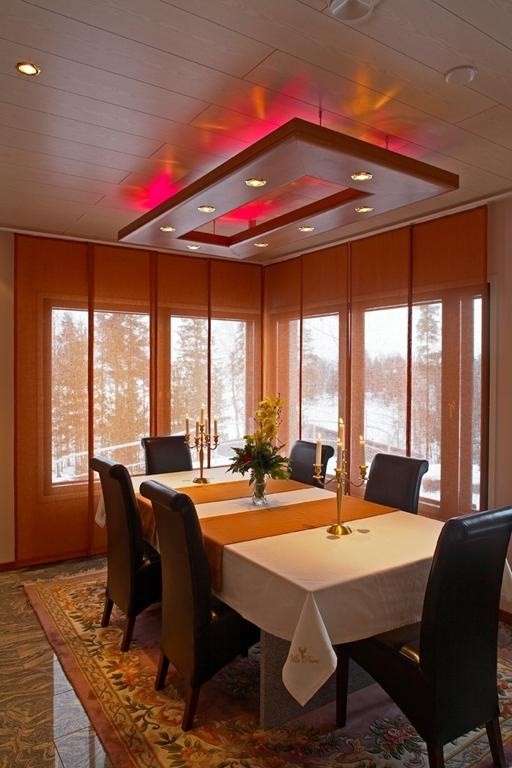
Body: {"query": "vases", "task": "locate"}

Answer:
[250,472,268,505]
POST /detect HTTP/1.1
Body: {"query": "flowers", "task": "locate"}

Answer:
[225,394,293,489]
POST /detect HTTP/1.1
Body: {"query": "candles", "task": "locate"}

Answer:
[182,404,220,436]
[313,419,365,472]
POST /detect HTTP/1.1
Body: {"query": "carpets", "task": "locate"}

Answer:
[25,564,512,768]
